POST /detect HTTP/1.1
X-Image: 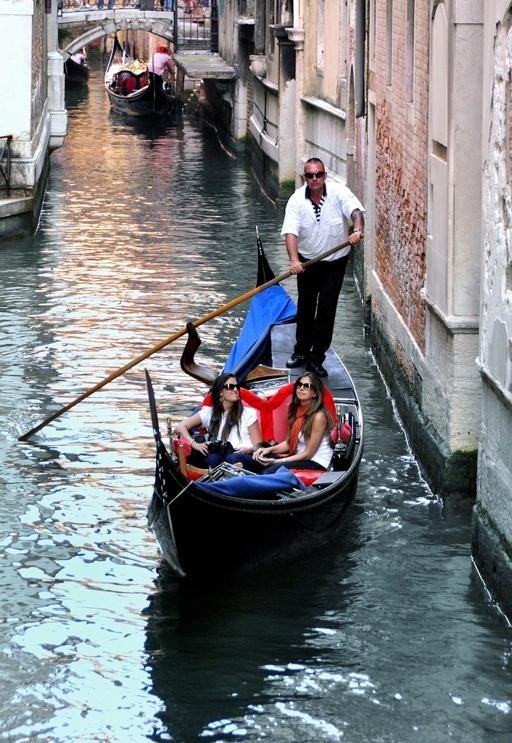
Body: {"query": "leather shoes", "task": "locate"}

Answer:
[306,362,328,377]
[286,351,308,369]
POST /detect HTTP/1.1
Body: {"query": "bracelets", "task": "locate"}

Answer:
[282,458,285,464]
[353,228,361,232]
[190,438,195,444]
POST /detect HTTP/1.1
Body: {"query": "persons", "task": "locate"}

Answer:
[173,373,264,474]
[147,44,174,90]
[279,157,366,378]
[63,0,210,23]
[252,372,335,476]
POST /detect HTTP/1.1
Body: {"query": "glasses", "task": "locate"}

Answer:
[304,171,326,179]
[224,383,240,391]
[296,381,314,391]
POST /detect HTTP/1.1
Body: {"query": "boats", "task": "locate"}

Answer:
[137,222,367,583]
[63,57,90,84]
[103,30,180,117]
[106,118,180,152]
[142,547,358,741]
[64,81,88,107]
